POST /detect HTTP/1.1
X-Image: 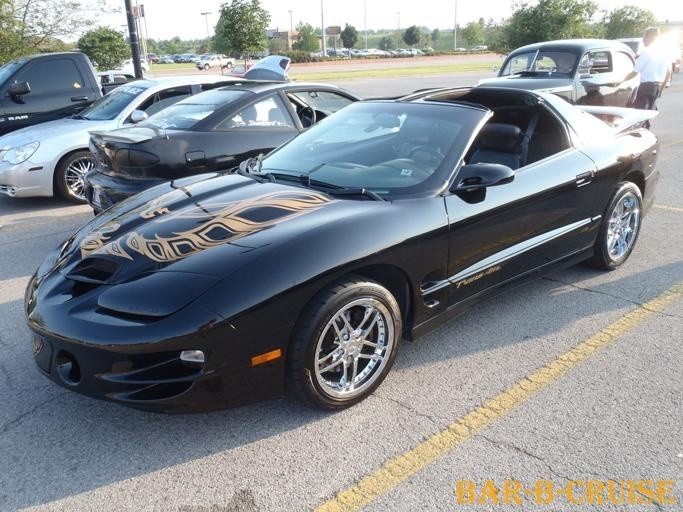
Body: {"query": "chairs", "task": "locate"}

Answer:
[398,118,524,183]
[241,105,290,129]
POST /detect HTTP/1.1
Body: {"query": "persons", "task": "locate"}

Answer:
[615,27,671,129]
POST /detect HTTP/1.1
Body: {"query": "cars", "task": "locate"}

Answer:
[472,45,487,51]
[473,38,646,109]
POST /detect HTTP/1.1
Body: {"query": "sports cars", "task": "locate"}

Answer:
[24,86,660,415]
